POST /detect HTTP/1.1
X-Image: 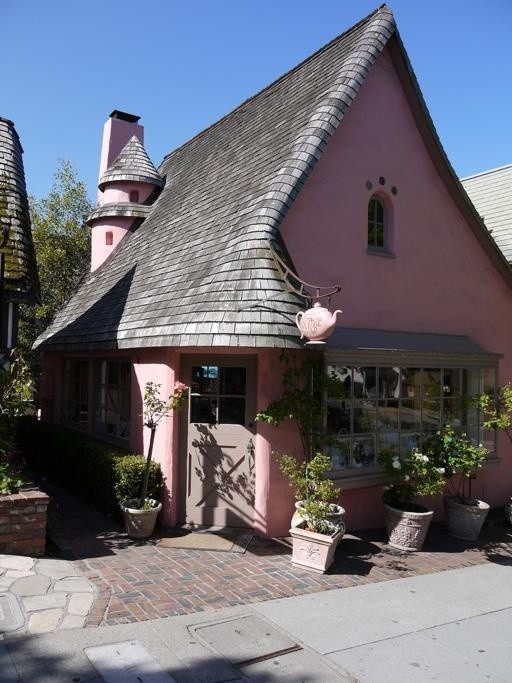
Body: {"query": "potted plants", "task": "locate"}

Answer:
[248,348,374,576]
[423,423,494,542]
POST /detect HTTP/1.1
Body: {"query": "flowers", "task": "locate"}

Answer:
[138,377,190,507]
[377,443,448,506]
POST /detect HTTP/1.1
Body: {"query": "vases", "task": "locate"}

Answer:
[384,504,434,552]
[118,498,161,539]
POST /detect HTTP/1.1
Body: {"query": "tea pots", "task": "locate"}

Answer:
[295,302,343,345]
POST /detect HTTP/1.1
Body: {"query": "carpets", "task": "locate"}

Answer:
[157,528,255,555]
